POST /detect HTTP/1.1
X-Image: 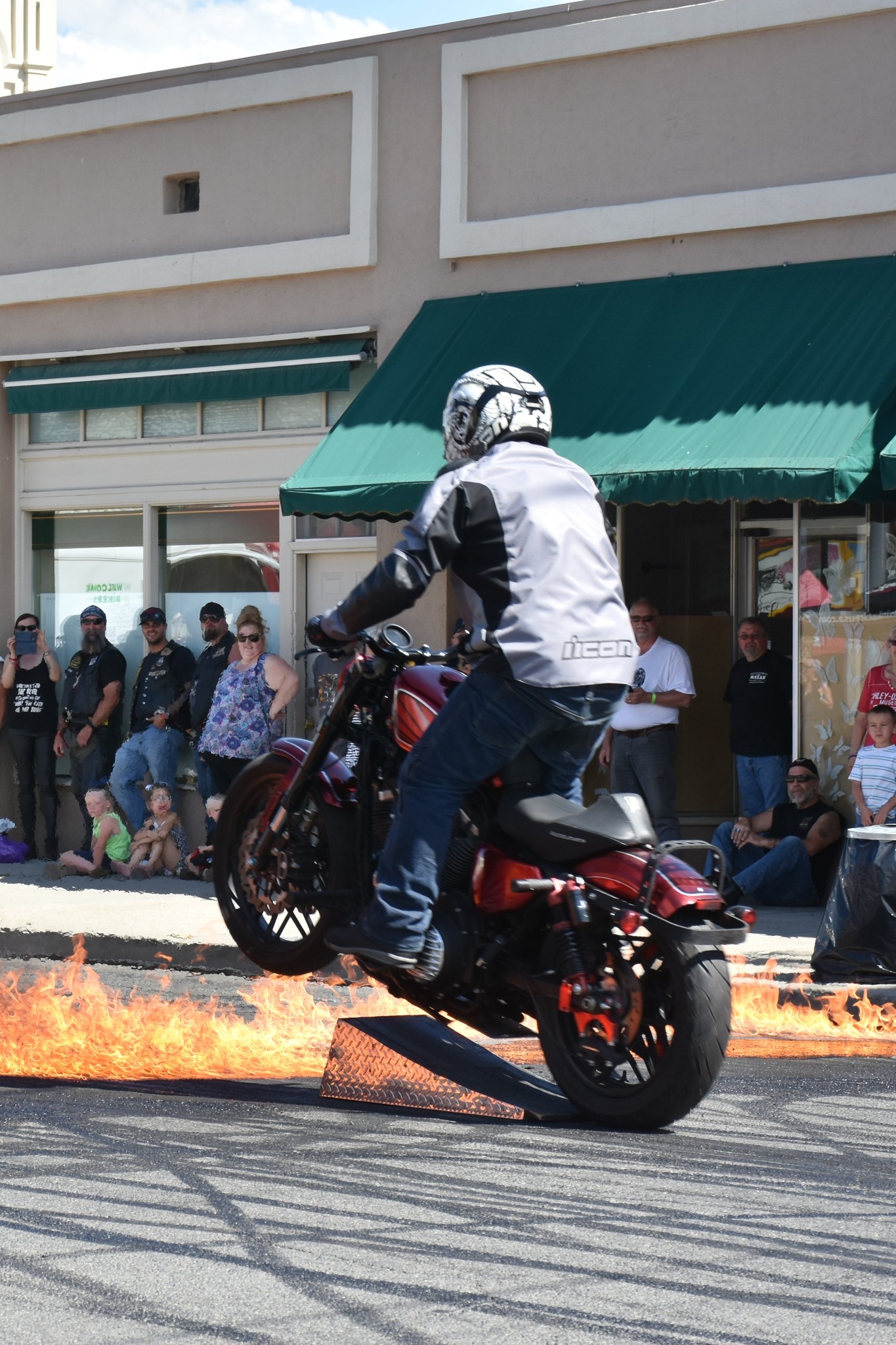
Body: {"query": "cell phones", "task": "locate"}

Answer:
[628,686,634,693]
[14,631,37,655]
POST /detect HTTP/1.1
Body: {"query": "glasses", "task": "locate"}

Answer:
[738,633,760,640]
[16,625,36,632]
[199,614,220,623]
[139,612,166,622]
[81,618,102,625]
[237,634,260,643]
[784,773,816,783]
[631,614,654,623]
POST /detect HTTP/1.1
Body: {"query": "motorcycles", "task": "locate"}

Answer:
[211,621,757,1134]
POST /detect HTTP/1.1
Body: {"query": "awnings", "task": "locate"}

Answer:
[3,336,377,416]
[275,253,896,521]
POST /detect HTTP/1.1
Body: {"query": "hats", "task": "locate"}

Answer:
[80,605,106,625]
[138,607,167,624]
[788,757,819,777]
[199,602,227,620]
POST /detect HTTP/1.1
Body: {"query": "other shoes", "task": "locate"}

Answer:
[89,866,112,878]
[320,916,419,972]
[44,862,78,878]
[111,857,152,881]
[178,865,213,882]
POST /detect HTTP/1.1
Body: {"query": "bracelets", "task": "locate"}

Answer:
[848,752,857,755]
[87,720,98,732]
[56,733,71,749]
[650,692,656,704]
[848,754,857,760]
[9,660,15,666]
[771,837,775,848]
[9,657,18,670]
[43,650,51,656]
[160,713,167,722]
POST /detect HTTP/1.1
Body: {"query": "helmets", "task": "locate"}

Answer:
[440,364,552,462]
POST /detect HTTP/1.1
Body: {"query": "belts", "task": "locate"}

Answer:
[617,723,673,739]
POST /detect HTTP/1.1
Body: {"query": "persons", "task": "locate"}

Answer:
[798,612,835,773]
[106,607,196,832]
[53,782,234,883]
[599,596,694,858]
[705,758,848,909]
[305,363,641,972]
[848,625,896,765]
[0,655,7,733]
[196,621,300,791]
[189,603,243,849]
[2,613,64,861]
[723,616,792,822]
[851,703,896,829]
[53,604,128,858]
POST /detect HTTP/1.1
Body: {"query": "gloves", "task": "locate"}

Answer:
[303,614,353,650]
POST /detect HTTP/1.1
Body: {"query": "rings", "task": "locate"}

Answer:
[736,829,740,832]
[629,700,632,703]
